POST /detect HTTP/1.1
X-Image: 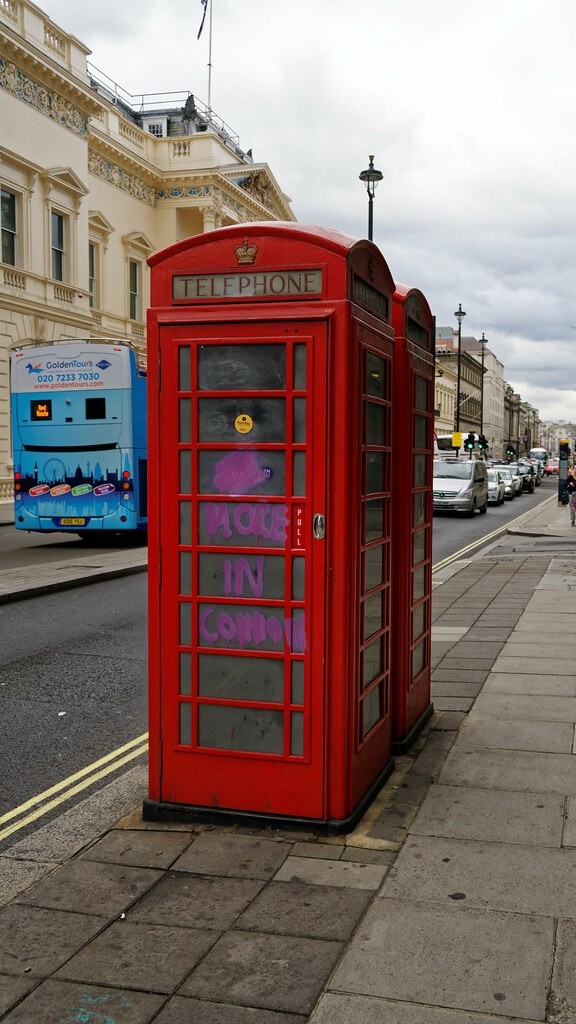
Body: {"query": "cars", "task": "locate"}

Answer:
[546,458,560,477]
[487,457,506,469]
[486,461,545,506]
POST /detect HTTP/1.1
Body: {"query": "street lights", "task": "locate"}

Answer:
[507,388,514,446]
[478,332,488,434]
[454,303,466,458]
[527,404,536,459]
[359,154,383,243]
[517,395,522,461]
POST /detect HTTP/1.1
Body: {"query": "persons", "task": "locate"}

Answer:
[566,467,576,527]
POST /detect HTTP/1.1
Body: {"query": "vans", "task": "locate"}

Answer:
[434,460,489,517]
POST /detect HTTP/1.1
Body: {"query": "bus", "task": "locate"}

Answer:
[530,448,548,466]
[11,337,148,544]
[434,433,483,461]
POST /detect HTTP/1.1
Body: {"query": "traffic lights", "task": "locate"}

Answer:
[464,434,475,452]
[478,435,489,451]
[506,446,516,455]
